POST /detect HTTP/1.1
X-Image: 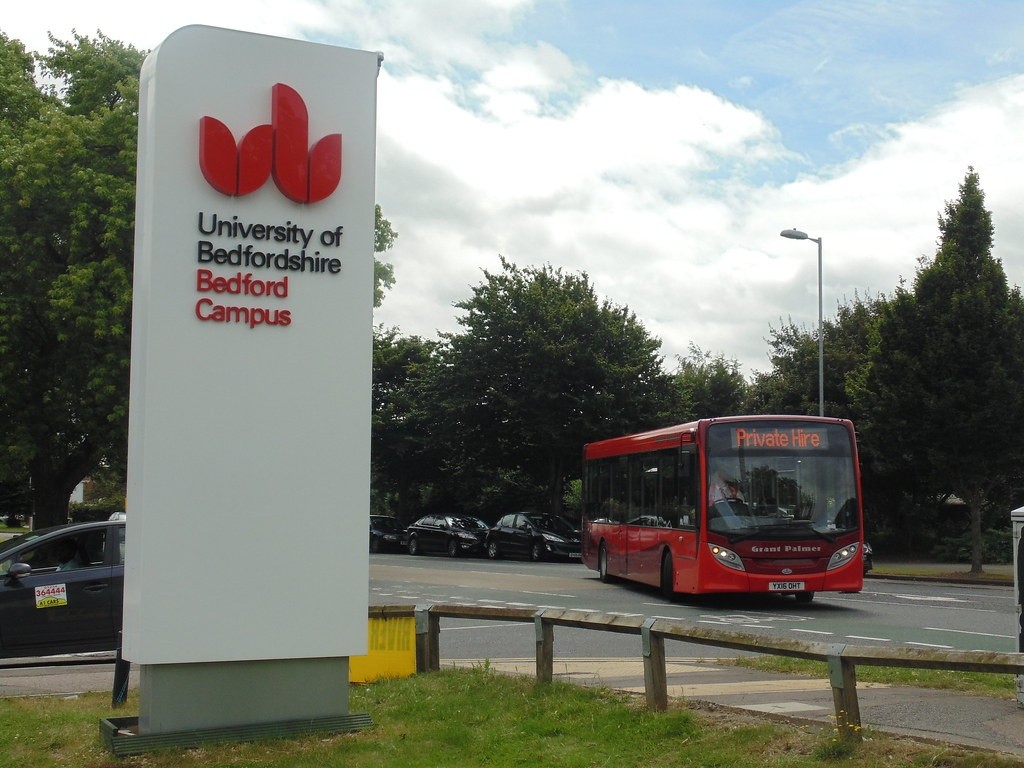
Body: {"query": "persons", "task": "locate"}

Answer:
[54,539,80,571]
[709,465,746,505]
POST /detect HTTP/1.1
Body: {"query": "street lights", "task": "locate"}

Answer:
[778,227,824,417]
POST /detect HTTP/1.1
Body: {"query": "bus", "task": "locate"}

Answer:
[581,414,863,605]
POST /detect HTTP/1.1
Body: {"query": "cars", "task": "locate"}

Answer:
[370,514,404,553]
[863,540,875,576]
[403,513,489,558]
[483,512,582,563]
[0,511,125,658]
